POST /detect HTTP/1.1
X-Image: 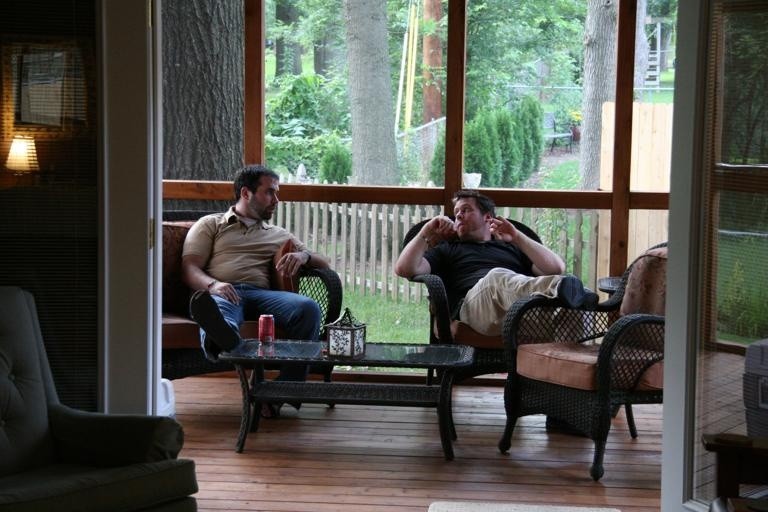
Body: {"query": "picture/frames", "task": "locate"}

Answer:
[3,40,76,141]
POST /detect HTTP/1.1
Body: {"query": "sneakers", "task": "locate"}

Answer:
[260,400,284,418]
[557,274,600,311]
[189,289,240,352]
[545,416,590,438]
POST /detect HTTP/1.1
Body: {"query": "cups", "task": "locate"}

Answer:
[464,173,482,188]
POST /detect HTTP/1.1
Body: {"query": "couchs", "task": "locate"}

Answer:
[160,211,342,380]
[0,287,199,510]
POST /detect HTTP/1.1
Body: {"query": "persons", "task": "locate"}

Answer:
[392,186,600,436]
[177,164,330,418]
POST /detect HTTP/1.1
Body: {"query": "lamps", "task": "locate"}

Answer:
[5,134,39,177]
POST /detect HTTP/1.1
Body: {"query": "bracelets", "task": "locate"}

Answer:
[206,279,217,291]
[418,231,431,249]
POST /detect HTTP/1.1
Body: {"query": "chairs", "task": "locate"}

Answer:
[698,429,767,510]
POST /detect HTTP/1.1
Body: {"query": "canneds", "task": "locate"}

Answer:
[258,345,275,357]
[258,313,275,345]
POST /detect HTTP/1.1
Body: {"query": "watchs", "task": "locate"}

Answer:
[301,249,312,265]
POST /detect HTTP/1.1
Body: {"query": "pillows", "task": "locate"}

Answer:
[272,239,299,294]
